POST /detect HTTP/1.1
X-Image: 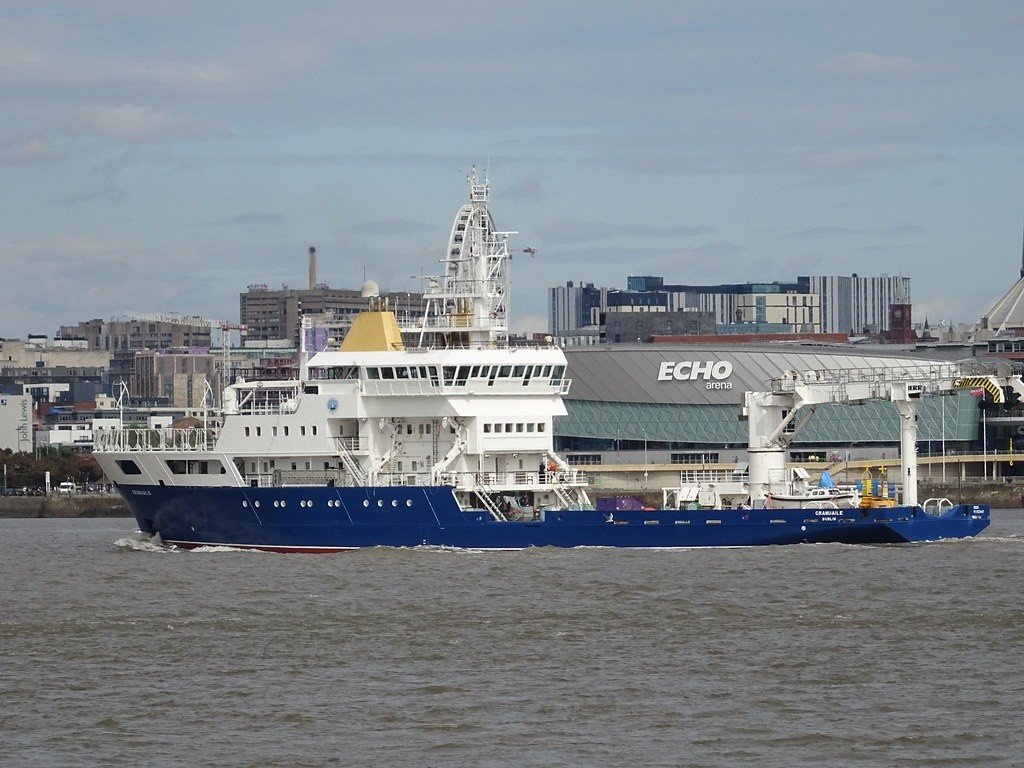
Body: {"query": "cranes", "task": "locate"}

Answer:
[121,310,249,412]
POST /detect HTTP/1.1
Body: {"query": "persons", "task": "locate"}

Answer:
[737,503,743,510]
[538,462,556,483]
[504,500,512,513]
[519,495,531,508]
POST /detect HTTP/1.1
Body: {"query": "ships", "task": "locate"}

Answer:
[91,161,1023,556]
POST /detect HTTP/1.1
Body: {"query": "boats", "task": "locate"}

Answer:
[764,487,862,509]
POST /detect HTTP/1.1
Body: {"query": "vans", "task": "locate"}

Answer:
[60,481,76,496]
